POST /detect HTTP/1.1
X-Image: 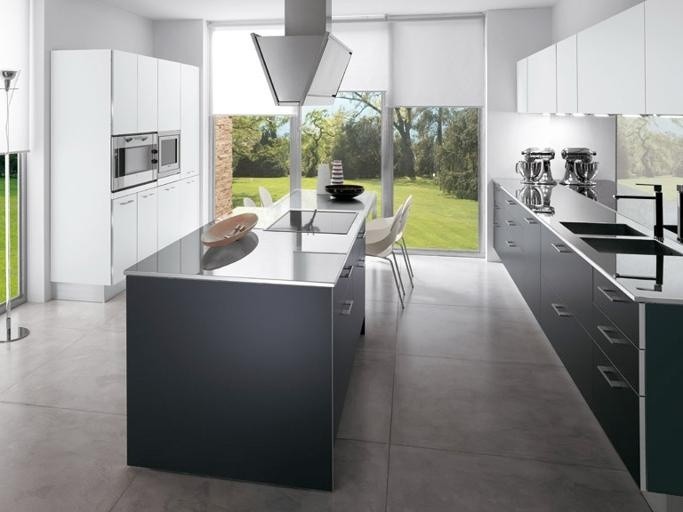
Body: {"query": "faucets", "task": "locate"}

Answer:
[613,252,664,293]
[613,183,664,237]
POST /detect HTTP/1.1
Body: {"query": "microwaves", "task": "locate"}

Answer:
[157,131,181,180]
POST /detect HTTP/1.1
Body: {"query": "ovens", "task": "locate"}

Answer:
[110,131,160,195]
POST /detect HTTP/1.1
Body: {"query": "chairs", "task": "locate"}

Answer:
[258,186,275,208]
[243,197,255,207]
[365,203,405,312]
[366,195,413,288]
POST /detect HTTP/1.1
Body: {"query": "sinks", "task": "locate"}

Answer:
[580,236,682,258]
[559,220,649,237]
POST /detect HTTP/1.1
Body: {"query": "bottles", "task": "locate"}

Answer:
[316,159,331,194]
[330,163,344,185]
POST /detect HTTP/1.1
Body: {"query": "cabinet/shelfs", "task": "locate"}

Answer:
[515,58,527,115]
[558,31,577,113]
[126,219,365,492]
[493,183,540,323]
[540,223,682,491]
[578,3,645,115]
[644,0,683,115]
[527,43,557,113]
[49,49,202,306]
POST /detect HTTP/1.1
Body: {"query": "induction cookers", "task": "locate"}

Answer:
[265,208,359,235]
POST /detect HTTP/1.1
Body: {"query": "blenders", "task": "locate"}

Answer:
[560,146,600,186]
[515,147,558,184]
[514,185,553,213]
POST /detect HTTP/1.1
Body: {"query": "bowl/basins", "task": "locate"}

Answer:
[326,185,364,200]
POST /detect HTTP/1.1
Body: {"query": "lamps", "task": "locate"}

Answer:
[0,65,29,342]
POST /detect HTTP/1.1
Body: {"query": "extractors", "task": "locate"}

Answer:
[245,1,354,108]
